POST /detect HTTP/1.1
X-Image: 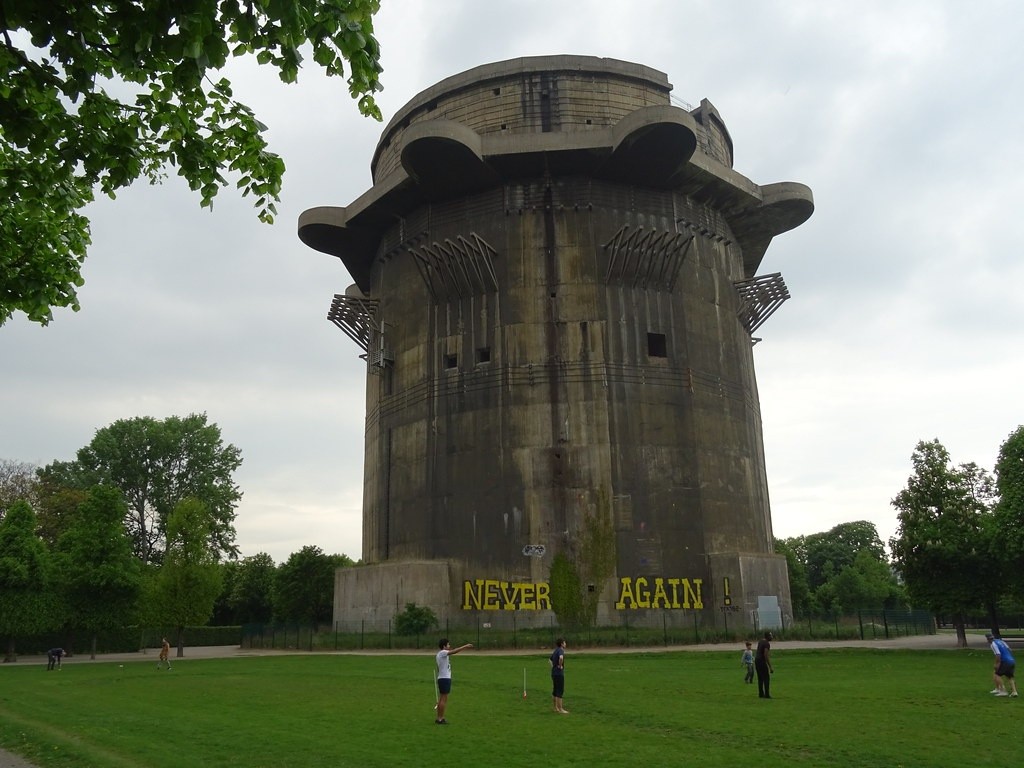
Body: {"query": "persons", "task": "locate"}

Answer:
[740,642,755,684]
[157,637,171,670]
[434,638,473,724]
[754,631,774,699]
[549,637,569,713]
[984,633,1018,697]
[47,648,67,671]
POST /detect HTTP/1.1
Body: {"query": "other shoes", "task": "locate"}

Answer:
[553,707,559,711]
[994,691,1008,696]
[1008,691,1018,697]
[765,695,775,699]
[157,667,159,670]
[558,709,569,714]
[168,667,171,670]
[758,694,765,698]
[990,688,999,694]
[750,682,754,684]
[744,678,748,684]
[435,718,448,724]
